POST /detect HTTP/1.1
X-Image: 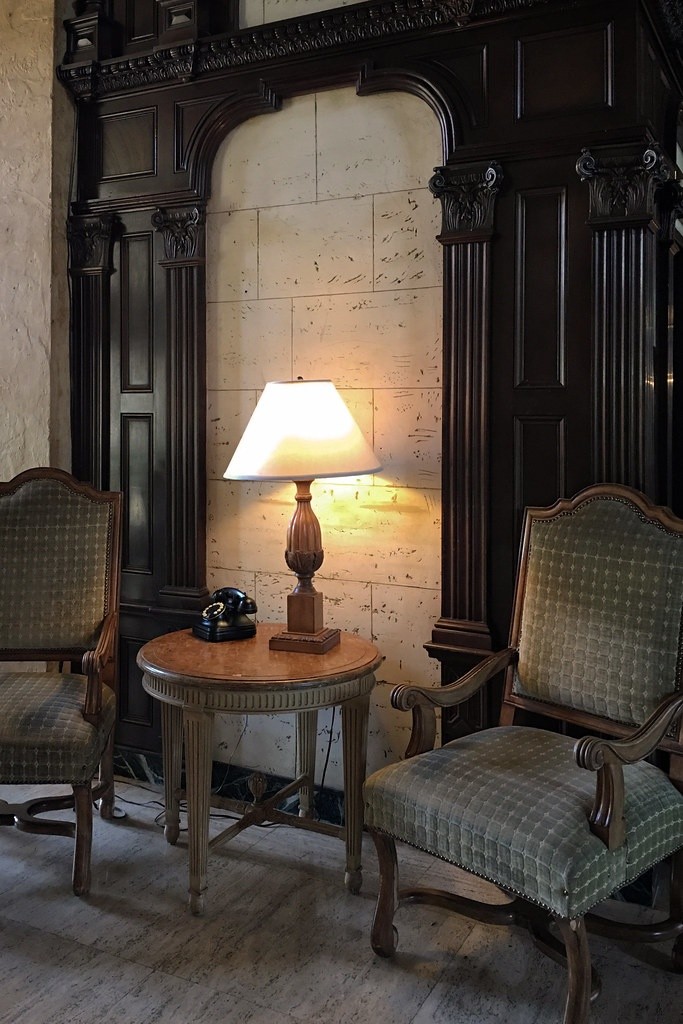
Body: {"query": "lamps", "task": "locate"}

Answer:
[220,379,385,656]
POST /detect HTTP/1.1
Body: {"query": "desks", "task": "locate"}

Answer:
[135,624,384,915]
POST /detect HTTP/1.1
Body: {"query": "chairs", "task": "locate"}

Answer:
[364,480,682,1023]
[0,468,125,896]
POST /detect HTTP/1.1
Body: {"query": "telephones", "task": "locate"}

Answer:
[191,587,257,644]
